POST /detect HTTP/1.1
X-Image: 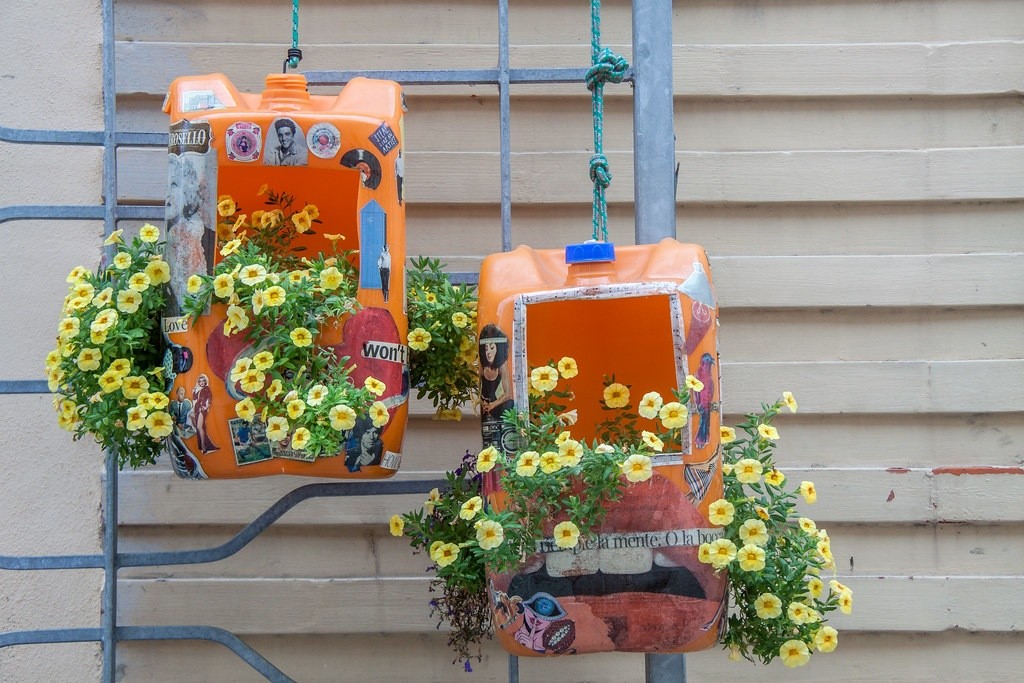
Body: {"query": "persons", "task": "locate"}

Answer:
[377,243,391,303]
[263,118,308,167]
[191,373,219,454]
[477,322,515,425]
[233,419,273,459]
[161,151,217,313]
[168,386,196,440]
[394,148,406,207]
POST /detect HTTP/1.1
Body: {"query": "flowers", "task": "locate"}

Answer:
[47,224,851,667]
